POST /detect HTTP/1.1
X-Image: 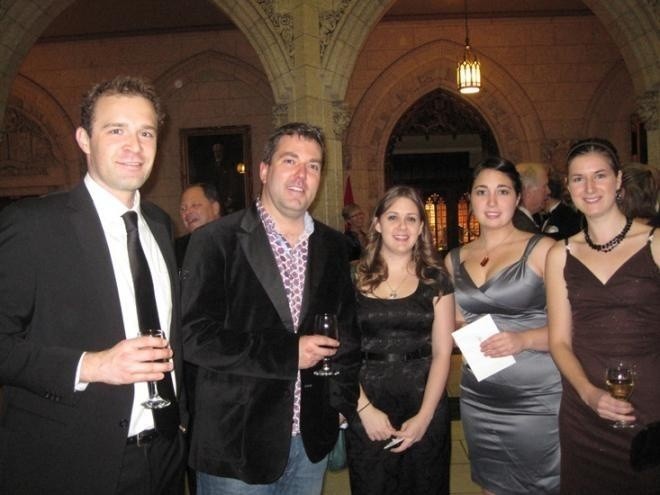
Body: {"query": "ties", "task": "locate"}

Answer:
[121,212,181,446]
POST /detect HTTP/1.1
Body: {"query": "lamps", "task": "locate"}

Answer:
[455,0,482,95]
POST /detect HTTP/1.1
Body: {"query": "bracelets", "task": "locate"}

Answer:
[358,401,371,414]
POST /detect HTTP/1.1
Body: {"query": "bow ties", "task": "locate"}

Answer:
[543,212,552,220]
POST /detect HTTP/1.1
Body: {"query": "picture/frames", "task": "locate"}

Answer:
[178,123,253,217]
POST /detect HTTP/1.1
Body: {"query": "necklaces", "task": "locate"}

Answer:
[480,228,514,267]
[386,273,409,297]
[583,220,632,253]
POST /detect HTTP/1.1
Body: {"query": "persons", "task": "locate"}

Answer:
[175,182,227,266]
[209,140,242,215]
[544,136,660,495]
[342,203,369,264]
[0,75,188,495]
[512,161,554,234]
[539,171,579,239]
[343,182,445,495]
[442,154,560,494]
[616,160,660,228]
[178,122,363,495]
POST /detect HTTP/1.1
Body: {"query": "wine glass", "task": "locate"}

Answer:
[137,328,172,409]
[604,368,637,430]
[313,313,342,376]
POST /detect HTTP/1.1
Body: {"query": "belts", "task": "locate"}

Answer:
[128,428,157,446]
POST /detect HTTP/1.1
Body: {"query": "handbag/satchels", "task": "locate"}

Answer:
[327,428,348,471]
